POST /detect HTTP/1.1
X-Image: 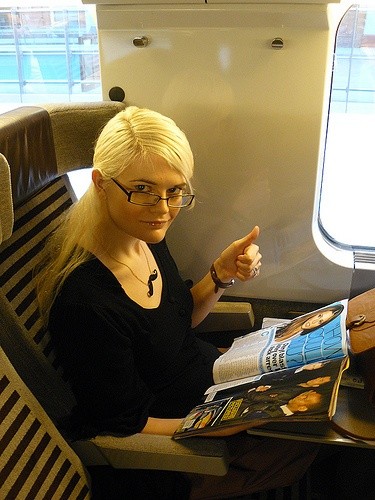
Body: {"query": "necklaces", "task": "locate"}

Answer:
[91,230,158,297]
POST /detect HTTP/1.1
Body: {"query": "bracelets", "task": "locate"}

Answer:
[209,263,234,287]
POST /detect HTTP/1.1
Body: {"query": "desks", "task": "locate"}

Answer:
[245,307,375,452]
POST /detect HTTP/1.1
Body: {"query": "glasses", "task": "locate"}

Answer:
[110,178,195,207]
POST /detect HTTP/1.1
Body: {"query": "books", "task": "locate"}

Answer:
[172,298,349,439]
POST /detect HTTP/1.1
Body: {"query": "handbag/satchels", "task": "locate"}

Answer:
[345,288,375,356]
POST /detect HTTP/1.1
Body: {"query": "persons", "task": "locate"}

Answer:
[241,360,332,418]
[274,304,344,342]
[38,106,323,500]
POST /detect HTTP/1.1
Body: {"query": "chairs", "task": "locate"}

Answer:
[0,107,256,500]
